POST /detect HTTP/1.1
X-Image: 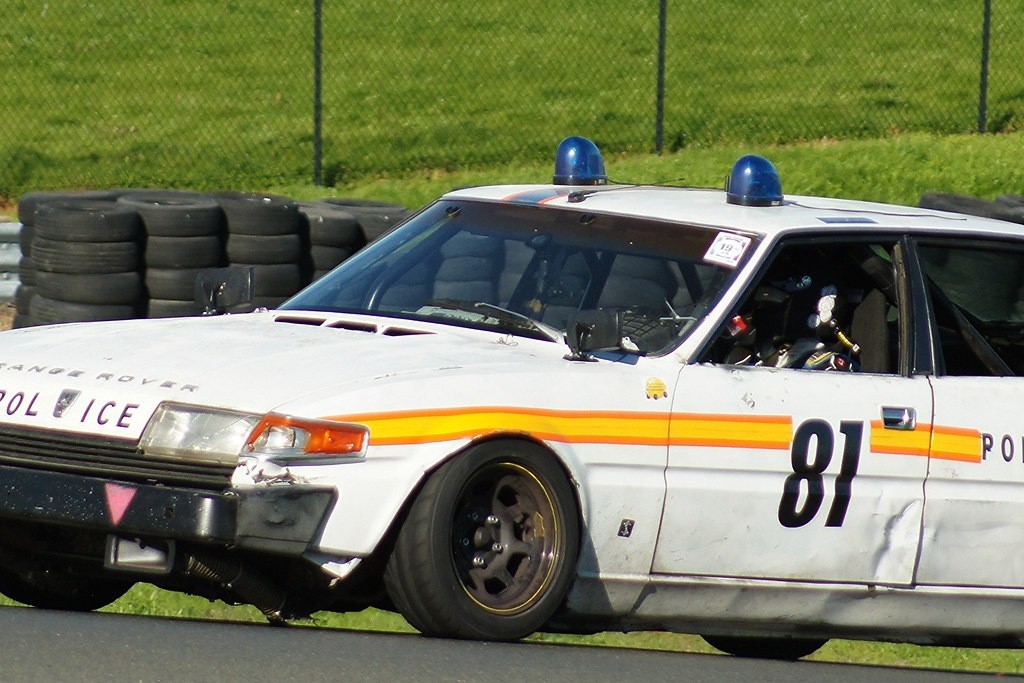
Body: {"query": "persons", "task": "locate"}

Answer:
[726,269,860,372]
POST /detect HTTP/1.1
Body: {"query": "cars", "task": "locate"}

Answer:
[0,134,1024,654]
